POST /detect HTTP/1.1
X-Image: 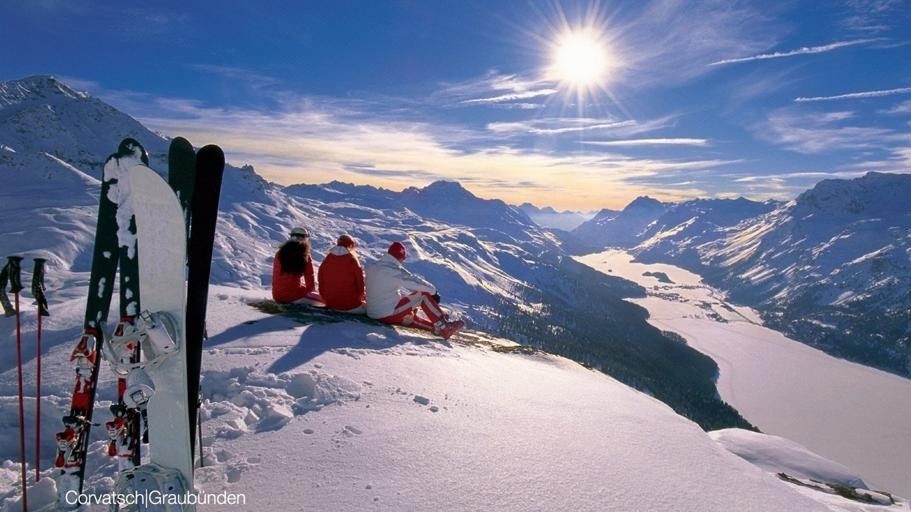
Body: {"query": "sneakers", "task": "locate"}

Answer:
[440,320,464,341]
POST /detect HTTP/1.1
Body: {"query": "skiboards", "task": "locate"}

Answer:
[54,137,225,511]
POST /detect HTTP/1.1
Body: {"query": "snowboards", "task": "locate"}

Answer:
[54,137,225,511]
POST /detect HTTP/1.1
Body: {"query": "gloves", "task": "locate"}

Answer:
[432,290,441,303]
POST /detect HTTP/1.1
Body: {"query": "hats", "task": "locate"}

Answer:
[290,227,308,241]
[337,235,354,248]
[388,241,406,260]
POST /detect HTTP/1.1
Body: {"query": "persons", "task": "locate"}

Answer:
[271,227,325,309]
[364,241,464,340]
[317,234,366,316]
